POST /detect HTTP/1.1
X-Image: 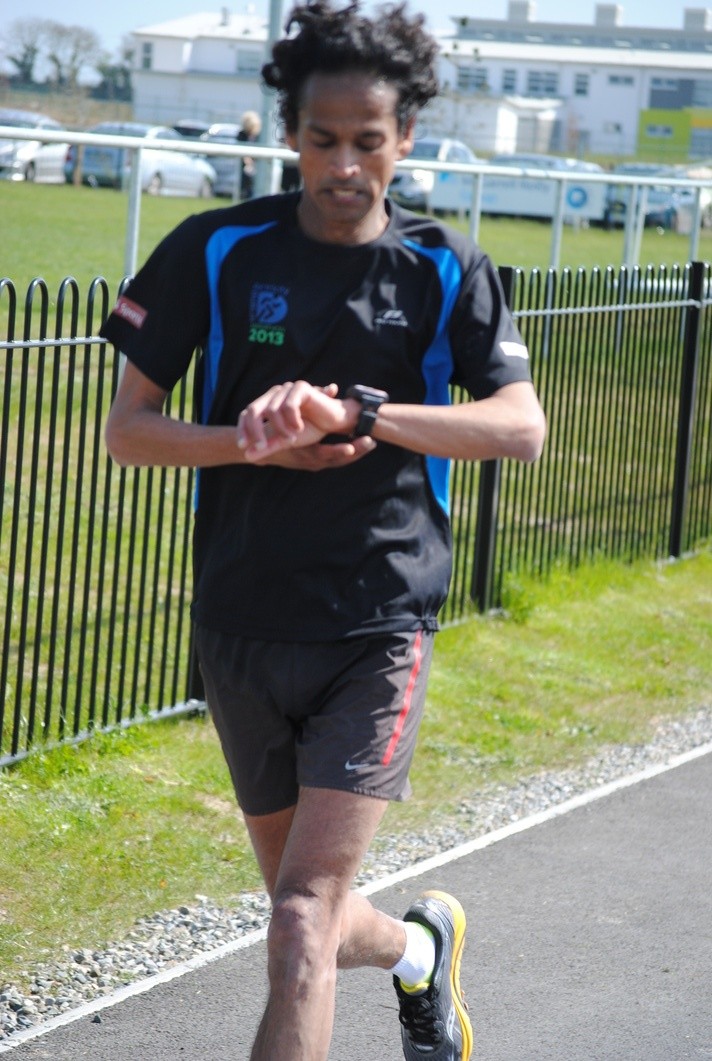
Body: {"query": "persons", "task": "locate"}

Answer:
[236,111,263,194]
[98,2,547,1061]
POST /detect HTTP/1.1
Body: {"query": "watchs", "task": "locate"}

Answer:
[346,383,390,438]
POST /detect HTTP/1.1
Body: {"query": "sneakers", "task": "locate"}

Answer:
[390,889,473,1059]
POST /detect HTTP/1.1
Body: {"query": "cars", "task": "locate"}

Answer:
[591,162,712,235]
[384,138,479,219]
[63,120,219,199]
[0,108,68,188]
[476,154,607,226]
[174,120,307,206]
